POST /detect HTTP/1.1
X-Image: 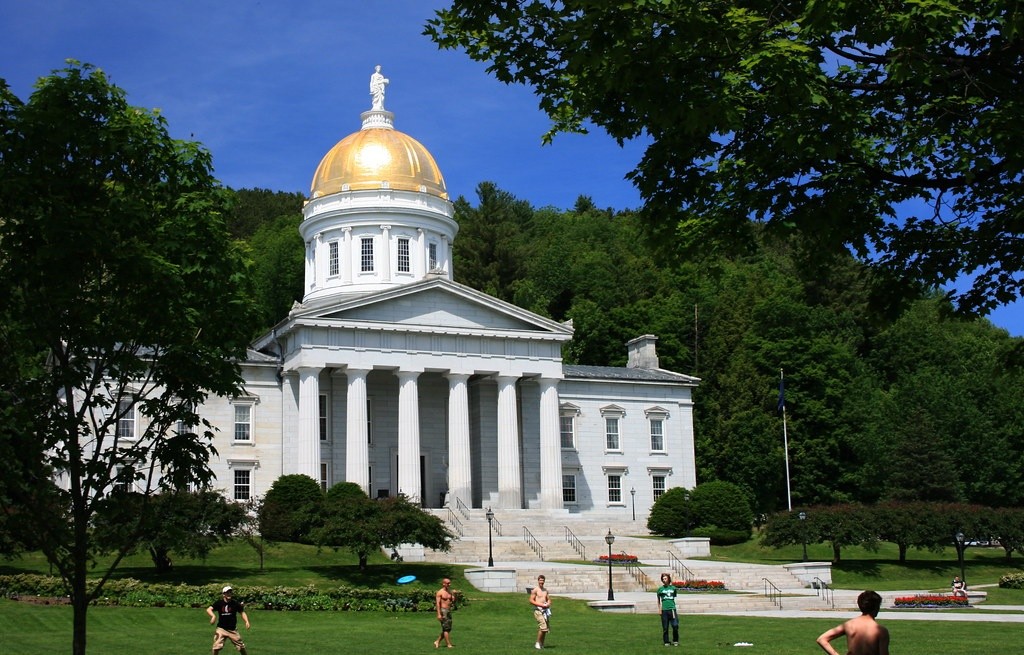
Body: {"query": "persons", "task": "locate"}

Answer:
[434,579,455,648]
[816,591,889,655]
[657,573,679,647]
[952,576,967,598]
[207,586,250,655]
[530,575,550,649]
[370,66,389,111]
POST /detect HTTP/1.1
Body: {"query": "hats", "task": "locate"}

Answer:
[222,586,232,594]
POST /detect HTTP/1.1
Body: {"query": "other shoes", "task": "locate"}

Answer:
[535,642,544,649]
[665,643,669,646]
[674,642,678,647]
